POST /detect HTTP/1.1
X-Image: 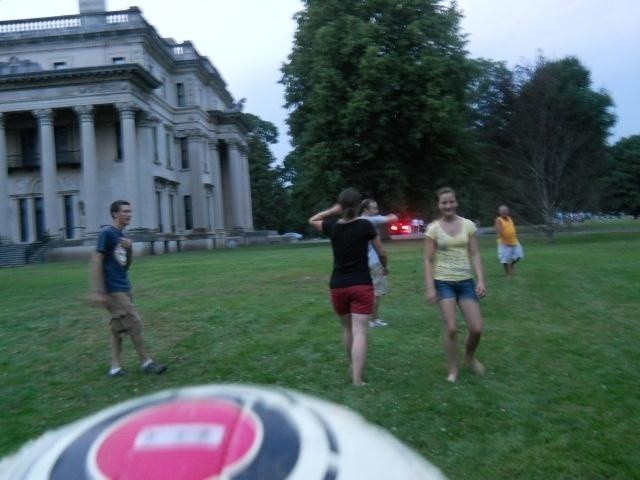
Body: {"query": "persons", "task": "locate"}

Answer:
[307,188,389,390]
[86,199,169,375]
[419,219,424,233]
[357,197,400,328]
[494,203,524,274]
[423,188,489,381]
[412,218,420,236]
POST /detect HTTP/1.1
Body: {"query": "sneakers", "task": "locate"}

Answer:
[142,358,168,375]
[109,368,129,377]
[368,316,390,328]
